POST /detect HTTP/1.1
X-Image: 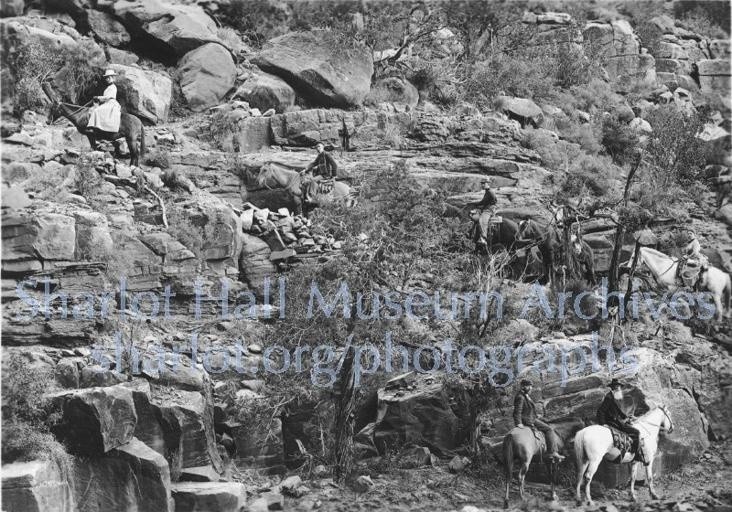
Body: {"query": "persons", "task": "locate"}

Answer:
[300,141,338,201]
[679,229,700,291]
[597,379,641,461]
[86,69,120,133]
[476,178,497,246]
[512,379,566,462]
[548,201,578,250]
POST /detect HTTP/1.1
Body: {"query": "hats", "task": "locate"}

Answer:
[103,70,117,77]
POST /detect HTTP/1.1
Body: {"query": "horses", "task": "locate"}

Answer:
[574,403,674,506]
[504,414,593,509]
[437,202,522,253]
[514,218,596,288]
[627,246,732,323]
[46,98,146,167]
[255,161,355,215]
